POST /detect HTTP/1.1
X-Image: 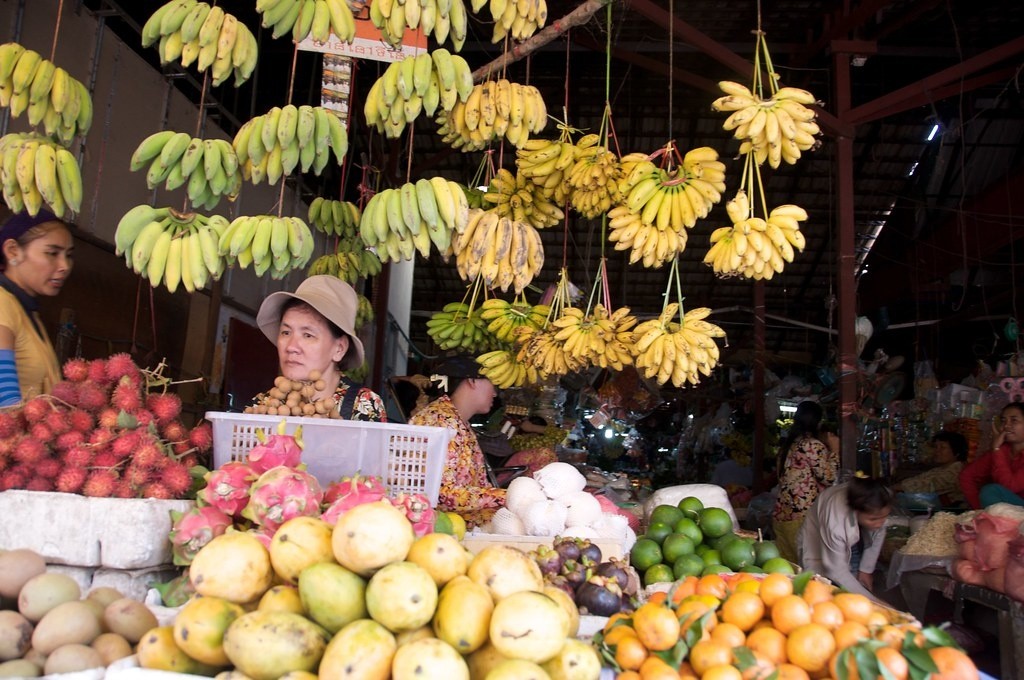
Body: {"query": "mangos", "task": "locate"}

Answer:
[135,500,602,679]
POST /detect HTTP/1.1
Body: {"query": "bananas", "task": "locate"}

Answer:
[0,0,824,386]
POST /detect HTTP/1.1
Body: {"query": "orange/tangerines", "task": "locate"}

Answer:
[596,573,980,680]
[509,425,568,451]
[631,496,795,586]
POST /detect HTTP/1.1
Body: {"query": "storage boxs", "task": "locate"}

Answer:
[1,488,197,570]
[205,410,449,509]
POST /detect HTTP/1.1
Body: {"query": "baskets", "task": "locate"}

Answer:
[205,411,457,513]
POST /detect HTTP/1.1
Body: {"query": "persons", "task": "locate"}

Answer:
[0,206,74,409]
[256,274,387,423]
[796,478,893,601]
[480,407,546,469]
[402,353,508,530]
[770,401,840,566]
[960,402,1024,509]
[891,431,968,494]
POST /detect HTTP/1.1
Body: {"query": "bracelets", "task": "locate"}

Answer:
[992,448,1000,451]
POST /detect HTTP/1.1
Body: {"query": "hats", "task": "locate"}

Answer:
[256,276,364,369]
